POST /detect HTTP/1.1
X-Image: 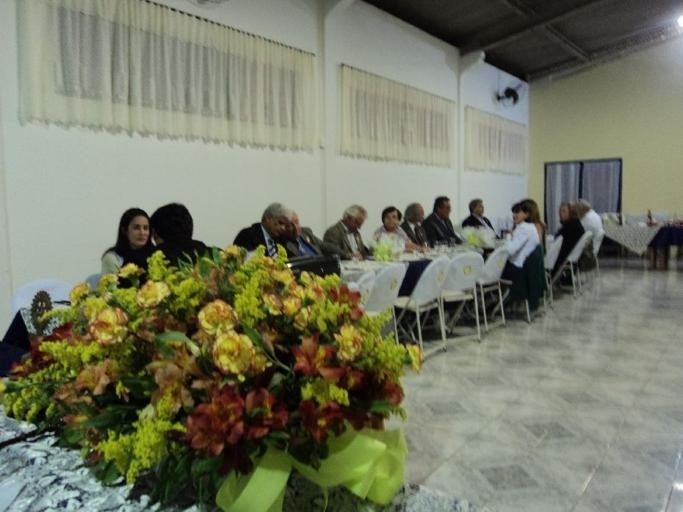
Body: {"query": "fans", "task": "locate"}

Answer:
[494,77,529,110]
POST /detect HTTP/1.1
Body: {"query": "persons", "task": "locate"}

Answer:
[275,206,352,278]
[478,202,541,318]
[101,208,158,293]
[460,199,501,241]
[418,195,463,245]
[145,202,228,276]
[567,198,606,271]
[522,199,547,247]
[370,206,428,257]
[398,201,432,251]
[232,201,293,274]
[547,200,586,284]
[321,205,374,264]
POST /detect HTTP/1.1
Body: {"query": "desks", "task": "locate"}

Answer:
[603,219,683,270]
[0,398,476,511]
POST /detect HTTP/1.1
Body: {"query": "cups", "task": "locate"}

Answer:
[419,238,457,255]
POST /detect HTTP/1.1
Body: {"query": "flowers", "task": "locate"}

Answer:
[0,246,429,512]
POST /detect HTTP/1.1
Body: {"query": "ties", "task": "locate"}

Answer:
[267,239,275,257]
